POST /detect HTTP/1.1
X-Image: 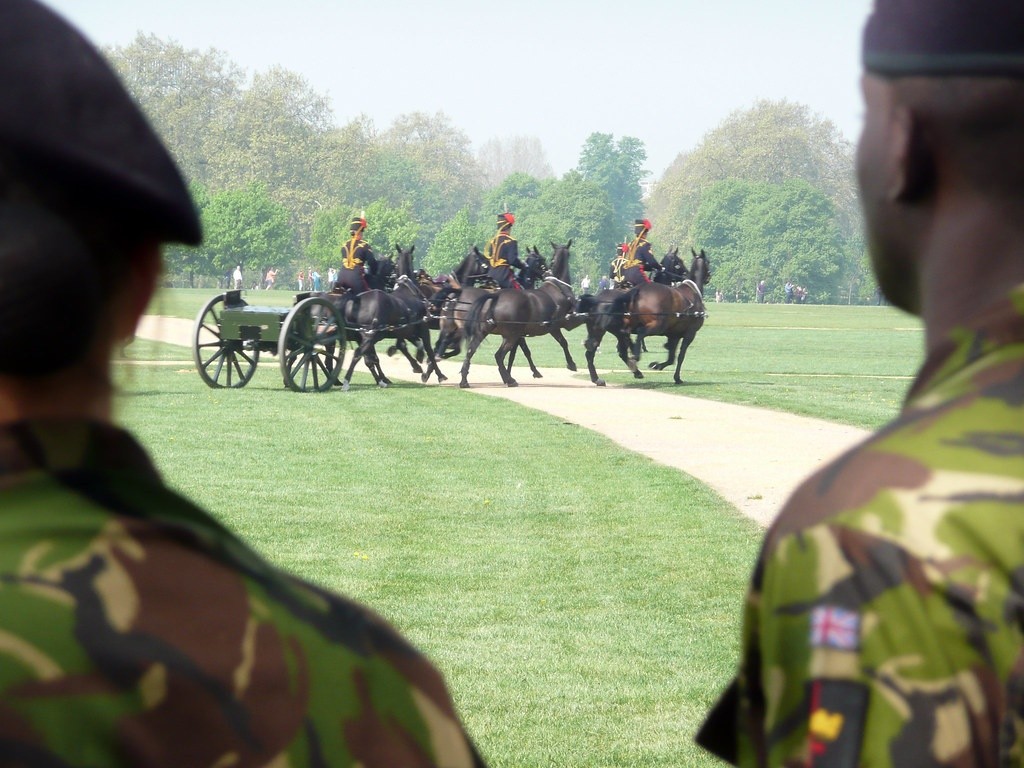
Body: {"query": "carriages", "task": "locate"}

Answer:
[194,237,712,396]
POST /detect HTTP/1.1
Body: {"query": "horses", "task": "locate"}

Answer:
[582,244,709,386]
[320,242,491,392]
[421,238,545,384]
[459,238,585,387]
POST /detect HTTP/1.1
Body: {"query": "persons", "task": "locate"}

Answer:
[695,1,1024,768]
[312,268,322,290]
[299,270,305,291]
[785,281,794,304]
[307,267,313,291]
[233,266,242,289]
[484,213,528,288]
[225,269,231,289]
[336,217,378,295]
[610,219,662,288]
[0,0,488,768]
[328,268,337,292]
[265,266,279,290]
[581,275,591,295]
[796,286,808,304]
[715,291,724,304]
[251,282,261,289]
[875,285,881,306]
[757,280,765,303]
[599,276,606,290]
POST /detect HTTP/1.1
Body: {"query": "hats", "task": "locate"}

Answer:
[616,244,629,254]
[863,0,1024,71]
[497,213,515,230]
[634,219,651,234]
[350,217,367,233]
[0,0,205,248]
